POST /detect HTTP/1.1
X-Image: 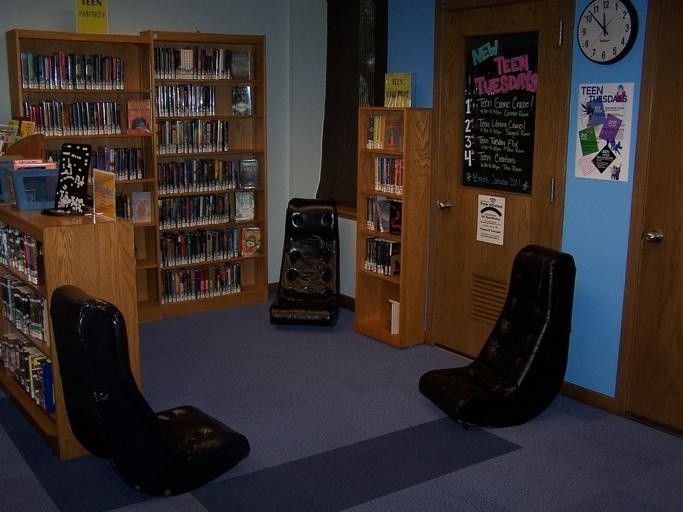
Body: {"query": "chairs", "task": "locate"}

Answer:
[419,245,576,427]
[268,199,341,327]
[50,282,250,496]
[577,0,640,64]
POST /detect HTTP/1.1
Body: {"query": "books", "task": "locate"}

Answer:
[0,124,18,156]
[0,219,55,415]
[7,51,154,216]
[363,110,400,277]
[154,44,263,304]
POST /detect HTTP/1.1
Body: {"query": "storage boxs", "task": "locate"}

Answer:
[0,160,59,210]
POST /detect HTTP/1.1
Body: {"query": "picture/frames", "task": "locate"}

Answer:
[74,0,110,34]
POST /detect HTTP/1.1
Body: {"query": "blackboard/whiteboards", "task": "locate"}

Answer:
[462,30,539,195]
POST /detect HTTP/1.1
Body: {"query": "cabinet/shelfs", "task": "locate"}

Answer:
[0,198,143,461]
[6,28,161,325]
[353,104,429,348]
[141,30,269,319]
